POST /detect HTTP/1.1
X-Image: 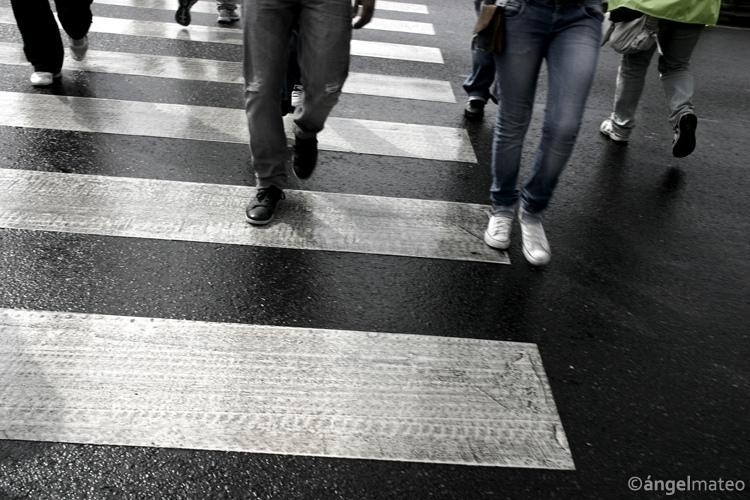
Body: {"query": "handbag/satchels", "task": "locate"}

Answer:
[602,12,660,53]
[472,6,506,53]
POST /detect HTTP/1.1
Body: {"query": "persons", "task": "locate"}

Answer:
[243,0,375,225]
[175,0,240,26]
[10,0,93,85]
[599,0,721,158]
[483,0,608,265]
[462,0,501,124]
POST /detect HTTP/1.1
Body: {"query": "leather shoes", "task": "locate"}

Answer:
[292,131,318,183]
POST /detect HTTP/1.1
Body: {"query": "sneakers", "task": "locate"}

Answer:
[29,71,62,85]
[68,36,89,60]
[485,211,515,249]
[176,1,196,25]
[671,111,697,158]
[246,187,282,225]
[517,208,551,267]
[216,8,240,23]
[291,83,306,108]
[599,119,632,142]
[465,99,486,116]
